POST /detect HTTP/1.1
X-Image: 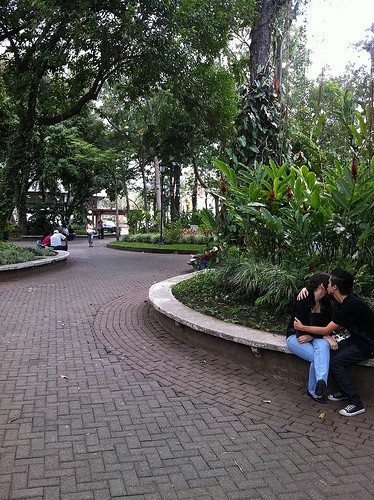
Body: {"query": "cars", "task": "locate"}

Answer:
[103,221,122,233]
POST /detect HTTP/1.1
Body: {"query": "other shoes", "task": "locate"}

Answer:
[307,391,327,403]
[315,380,326,396]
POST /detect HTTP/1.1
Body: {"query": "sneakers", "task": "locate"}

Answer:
[339,404,366,416]
[328,392,348,401]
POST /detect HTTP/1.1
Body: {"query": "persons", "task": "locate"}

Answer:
[61,224,77,241]
[41,231,51,247]
[86,220,94,247]
[294,268,374,417]
[98,219,105,239]
[50,229,68,251]
[286,272,339,404]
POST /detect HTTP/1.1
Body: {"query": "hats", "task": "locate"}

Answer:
[54,230,59,233]
